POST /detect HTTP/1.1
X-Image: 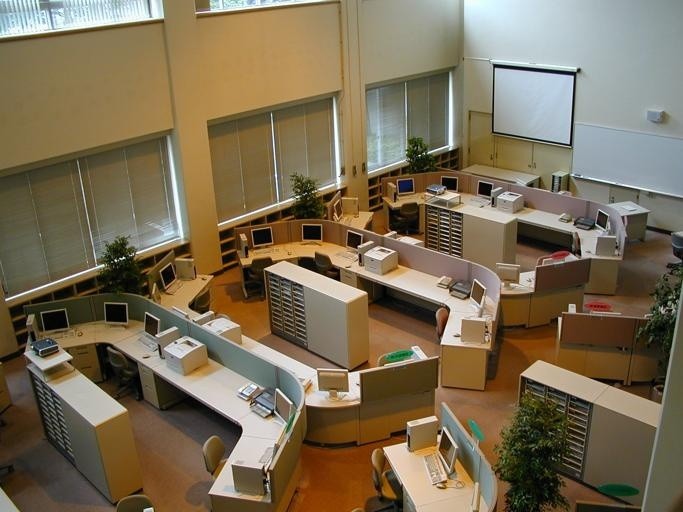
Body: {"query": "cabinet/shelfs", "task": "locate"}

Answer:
[517,360,661,506]
[368,146,459,211]
[8,241,191,350]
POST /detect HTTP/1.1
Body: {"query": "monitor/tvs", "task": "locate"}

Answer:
[346,230,363,251]
[470,278,487,309]
[495,263,521,291]
[273,423,288,453]
[302,224,323,246]
[472,482,480,512]
[317,368,349,400]
[334,199,343,221]
[103,302,129,329]
[40,308,69,334]
[251,226,273,248]
[159,262,177,290]
[436,426,459,473]
[145,312,160,338]
[396,178,415,195]
[272,387,294,427]
[477,180,494,198]
[441,176,458,192]
[595,209,610,236]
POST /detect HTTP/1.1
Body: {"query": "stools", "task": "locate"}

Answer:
[666,231,682,275]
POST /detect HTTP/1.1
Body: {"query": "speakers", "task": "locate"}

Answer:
[646,108,664,122]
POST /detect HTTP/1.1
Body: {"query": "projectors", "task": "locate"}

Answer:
[29,337,59,357]
[425,183,447,195]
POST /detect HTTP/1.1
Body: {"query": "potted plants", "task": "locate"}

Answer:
[634,264,682,403]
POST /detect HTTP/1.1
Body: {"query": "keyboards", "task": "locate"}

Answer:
[257,446,274,466]
[166,280,183,295]
[422,452,447,485]
[136,335,158,352]
[47,330,76,341]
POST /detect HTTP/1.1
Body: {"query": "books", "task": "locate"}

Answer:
[236,382,263,400]
[437,275,453,289]
[558,212,573,223]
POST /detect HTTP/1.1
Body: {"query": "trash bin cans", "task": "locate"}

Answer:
[671,231,683,259]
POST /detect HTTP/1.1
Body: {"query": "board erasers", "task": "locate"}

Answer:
[575,174,581,176]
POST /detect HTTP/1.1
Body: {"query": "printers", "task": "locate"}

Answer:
[448,280,472,299]
[363,246,398,275]
[164,336,207,376]
[496,191,524,214]
[574,216,595,230]
[201,318,243,346]
[249,388,275,418]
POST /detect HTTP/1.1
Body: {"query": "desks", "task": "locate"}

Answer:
[23,171,627,512]
[609,200,651,243]
[461,163,540,187]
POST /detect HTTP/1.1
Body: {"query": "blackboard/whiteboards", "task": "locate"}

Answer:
[570,121,683,199]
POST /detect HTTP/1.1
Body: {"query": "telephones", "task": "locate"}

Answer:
[297,377,313,393]
[236,382,259,401]
[437,276,452,289]
[558,213,572,223]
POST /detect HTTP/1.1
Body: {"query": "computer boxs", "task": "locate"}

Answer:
[25,313,39,344]
[230,458,266,495]
[406,415,438,452]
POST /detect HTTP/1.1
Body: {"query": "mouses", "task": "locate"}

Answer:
[142,354,150,358]
[77,331,82,337]
[436,483,446,489]
